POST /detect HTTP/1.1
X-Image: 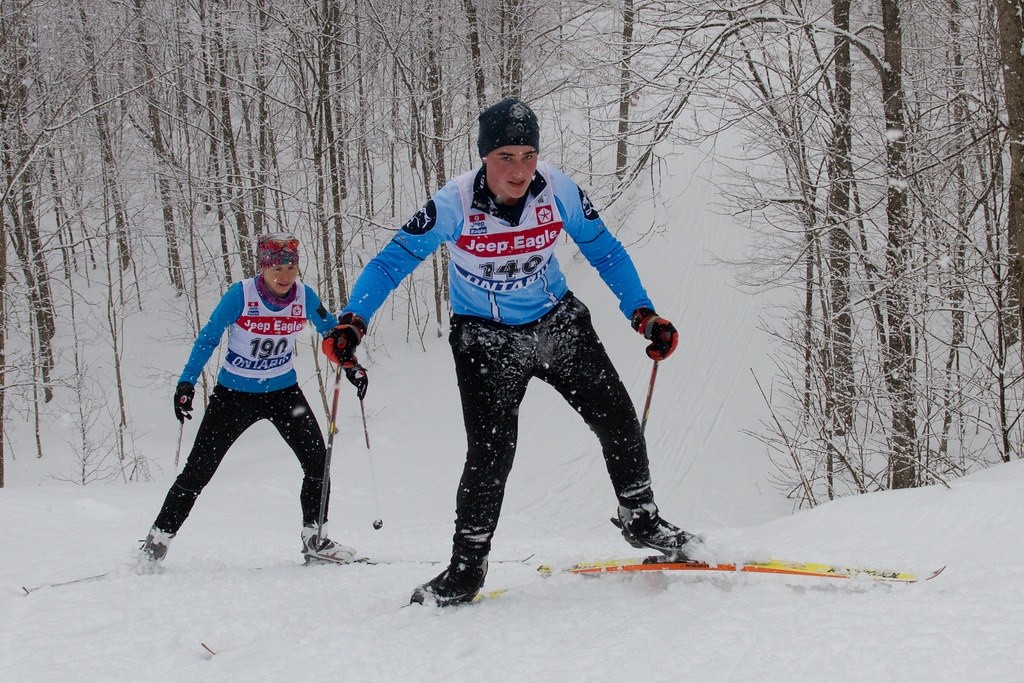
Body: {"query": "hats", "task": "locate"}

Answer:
[257,232,299,270]
[478,99,540,158]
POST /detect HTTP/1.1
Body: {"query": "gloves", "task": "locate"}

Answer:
[174,382,195,424]
[344,362,369,400]
[322,313,367,368]
[630,308,679,361]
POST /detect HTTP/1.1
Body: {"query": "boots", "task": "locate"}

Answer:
[136,525,176,574]
[409,536,490,606]
[611,493,703,560]
[300,521,357,564]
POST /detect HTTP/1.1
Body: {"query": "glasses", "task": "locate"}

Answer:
[264,240,300,249]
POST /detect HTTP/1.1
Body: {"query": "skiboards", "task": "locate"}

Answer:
[23,555,947,657]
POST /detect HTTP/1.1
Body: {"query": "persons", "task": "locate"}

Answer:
[136,233,369,563]
[314,97,704,610]
